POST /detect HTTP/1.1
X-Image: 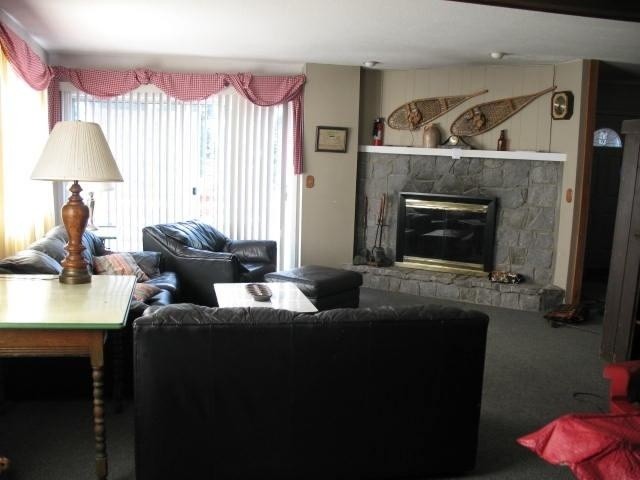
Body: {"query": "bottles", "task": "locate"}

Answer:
[498,129,507,151]
[423,124,441,148]
[372,118,383,146]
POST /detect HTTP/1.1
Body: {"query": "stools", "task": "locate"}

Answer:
[265,262,363,311]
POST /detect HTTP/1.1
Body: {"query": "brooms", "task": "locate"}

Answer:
[354,196,370,265]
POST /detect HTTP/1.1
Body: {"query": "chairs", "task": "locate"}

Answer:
[142,218,277,307]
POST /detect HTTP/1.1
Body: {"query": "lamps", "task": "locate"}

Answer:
[28,118,125,285]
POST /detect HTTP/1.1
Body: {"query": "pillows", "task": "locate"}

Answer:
[91,251,164,310]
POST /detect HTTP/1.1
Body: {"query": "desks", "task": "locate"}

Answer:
[0,271,139,480]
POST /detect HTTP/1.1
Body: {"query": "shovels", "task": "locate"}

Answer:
[358,196,371,265]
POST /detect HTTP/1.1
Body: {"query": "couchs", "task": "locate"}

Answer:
[1,221,177,399]
[129,299,490,479]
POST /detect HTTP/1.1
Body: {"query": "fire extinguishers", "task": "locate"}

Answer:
[372,117,384,146]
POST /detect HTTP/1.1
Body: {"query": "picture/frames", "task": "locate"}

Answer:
[314,125,349,153]
